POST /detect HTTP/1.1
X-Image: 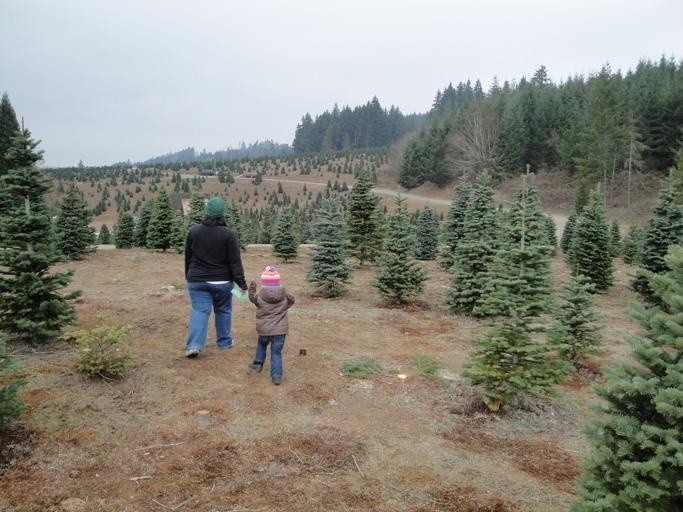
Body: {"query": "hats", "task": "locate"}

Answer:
[207,199,224,217]
[260,266,281,287]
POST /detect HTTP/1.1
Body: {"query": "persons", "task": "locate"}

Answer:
[249,266,295,385]
[183,197,249,358]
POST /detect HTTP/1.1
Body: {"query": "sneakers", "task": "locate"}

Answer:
[249,360,280,384]
[185,348,200,356]
